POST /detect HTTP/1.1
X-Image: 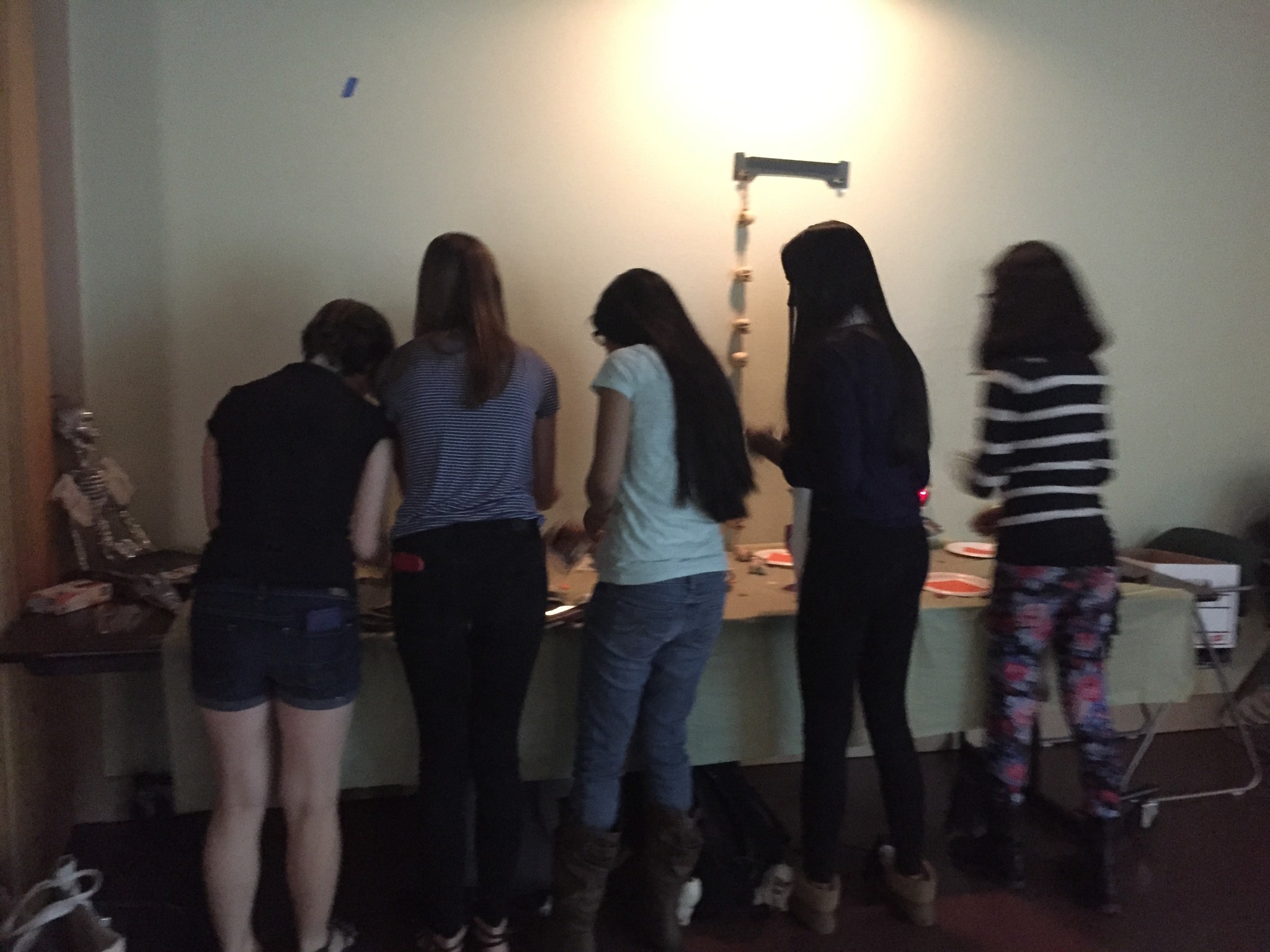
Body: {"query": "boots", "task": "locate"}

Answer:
[951,783,1029,891]
[624,807,703,952]
[1059,813,1119,903]
[526,798,622,952]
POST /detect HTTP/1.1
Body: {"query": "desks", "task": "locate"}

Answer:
[1,530,1192,907]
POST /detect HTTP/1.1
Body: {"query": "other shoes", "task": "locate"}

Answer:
[785,870,839,934]
[874,849,937,929]
[416,912,508,952]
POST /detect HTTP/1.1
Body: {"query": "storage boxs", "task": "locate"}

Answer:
[1111,546,1241,653]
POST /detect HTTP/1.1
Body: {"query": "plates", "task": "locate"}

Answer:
[944,542,998,558]
[753,548,794,566]
[921,573,993,596]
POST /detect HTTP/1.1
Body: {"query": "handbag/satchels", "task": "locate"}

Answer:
[0,868,124,952]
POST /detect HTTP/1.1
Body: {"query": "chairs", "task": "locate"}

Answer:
[1108,527,1265,806]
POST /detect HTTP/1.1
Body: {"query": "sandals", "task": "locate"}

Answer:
[310,923,360,952]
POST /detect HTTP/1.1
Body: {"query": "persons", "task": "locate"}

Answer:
[742,218,936,934]
[369,231,563,951]
[947,235,1132,904]
[549,264,758,950]
[191,299,399,951]
